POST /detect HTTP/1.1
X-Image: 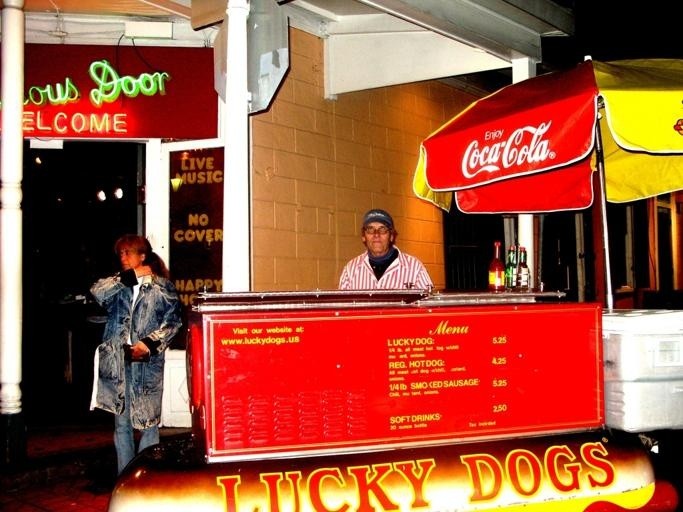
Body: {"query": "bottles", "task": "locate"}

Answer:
[488,241,528,293]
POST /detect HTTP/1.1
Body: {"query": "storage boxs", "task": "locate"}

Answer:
[604,381,683,434]
[602,308,683,382]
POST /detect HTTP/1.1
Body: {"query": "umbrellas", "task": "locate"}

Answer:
[406,50,683,313]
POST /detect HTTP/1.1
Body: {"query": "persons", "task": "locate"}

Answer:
[338,206,435,293]
[86,232,184,476]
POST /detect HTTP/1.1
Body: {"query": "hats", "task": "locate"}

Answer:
[363,209,393,229]
